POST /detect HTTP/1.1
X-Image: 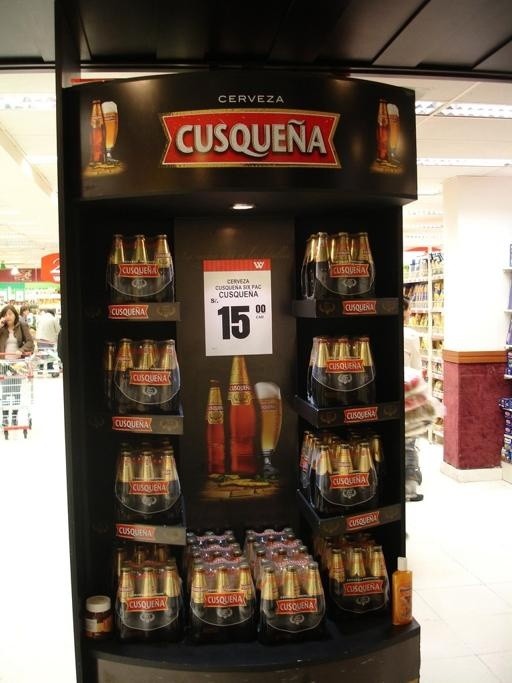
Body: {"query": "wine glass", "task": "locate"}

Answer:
[386,103,400,163]
[101,101,118,162]
[253,382,283,474]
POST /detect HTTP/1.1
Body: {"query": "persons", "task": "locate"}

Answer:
[403,296,426,375]
[1,302,36,425]
[17,303,61,378]
[405,435,425,501]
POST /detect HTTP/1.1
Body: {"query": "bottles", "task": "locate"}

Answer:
[376,98,389,161]
[204,377,224,476]
[109,527,392,643]
[227,355,258,476]
[391,556,414,626]
[82,595,114,640]
[298,230,385,514]
[104,231,186,526]
[88,99,106,165]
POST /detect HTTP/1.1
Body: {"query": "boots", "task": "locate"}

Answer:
[3,410,19,426]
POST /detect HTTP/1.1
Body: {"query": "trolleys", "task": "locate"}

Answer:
[0,352,33,439]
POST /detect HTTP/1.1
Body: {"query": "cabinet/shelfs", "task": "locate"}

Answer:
[499,268,512,483]
[404,245,446,444]
